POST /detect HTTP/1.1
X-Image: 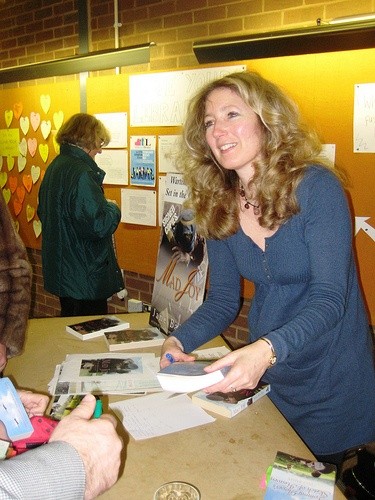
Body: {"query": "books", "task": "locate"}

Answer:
[156,360,232,393]
[104,327,166,351]
[66,316,130,341]
[263,451,336,500]
[191,382,271,418]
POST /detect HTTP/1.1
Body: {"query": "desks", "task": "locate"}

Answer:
[0,313,348,500]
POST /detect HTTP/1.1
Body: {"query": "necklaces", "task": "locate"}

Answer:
[240,184,260,215]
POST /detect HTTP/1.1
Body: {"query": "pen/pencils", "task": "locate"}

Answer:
[93,396,104,419]
[166,354,175,363]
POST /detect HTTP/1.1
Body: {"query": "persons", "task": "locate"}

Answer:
[131,167,154,180]
[0,389,124,500]
[0,186,33,373]
[37,113,125,318]
[161,72,375,473]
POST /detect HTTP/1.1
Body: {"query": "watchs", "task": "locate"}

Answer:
[260,337,277,368]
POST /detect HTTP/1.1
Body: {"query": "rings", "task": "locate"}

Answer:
[230,385,236,393]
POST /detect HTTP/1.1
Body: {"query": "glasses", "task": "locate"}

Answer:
[100,138,105,146]
